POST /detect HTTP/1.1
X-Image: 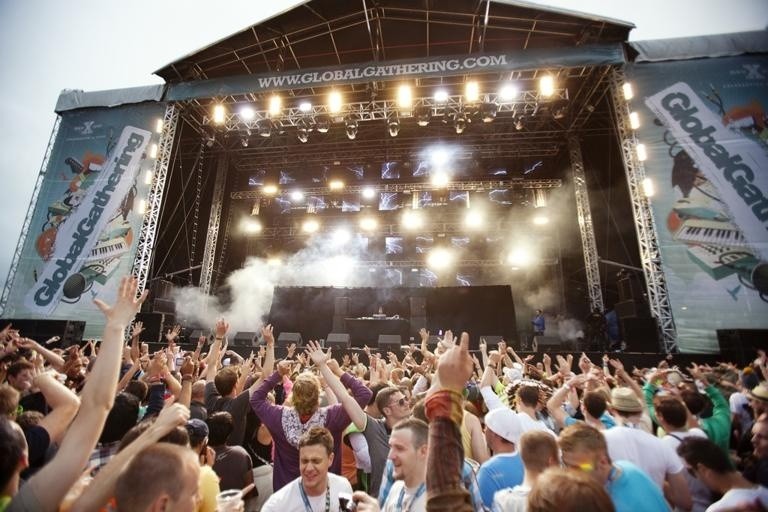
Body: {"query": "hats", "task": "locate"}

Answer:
[187,420,208,446]
[746,386,768,403]
[485,408,521,443]
[606,387,643,413]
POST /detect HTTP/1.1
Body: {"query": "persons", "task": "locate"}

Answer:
[1,275,768,512]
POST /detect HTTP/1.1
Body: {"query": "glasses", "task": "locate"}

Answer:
[390,396,408,407]
